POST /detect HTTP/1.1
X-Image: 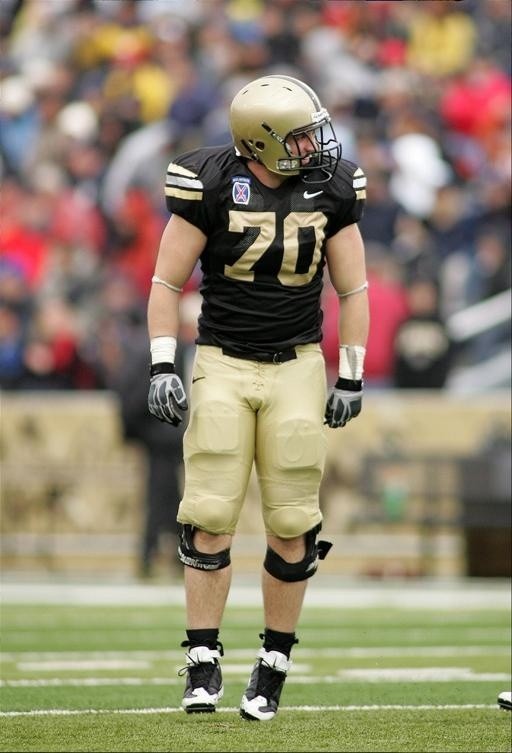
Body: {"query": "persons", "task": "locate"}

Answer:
[142,73,371,722]
[114,289,207,585]
[0,0,512,393]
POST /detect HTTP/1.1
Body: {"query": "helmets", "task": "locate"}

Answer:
[229,75,331,176]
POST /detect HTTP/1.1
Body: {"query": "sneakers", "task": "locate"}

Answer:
[240,647,292,721]
[181,645,224,714]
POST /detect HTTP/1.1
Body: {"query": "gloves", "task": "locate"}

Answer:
[145,362,188,427]
[322,376,364,429]
